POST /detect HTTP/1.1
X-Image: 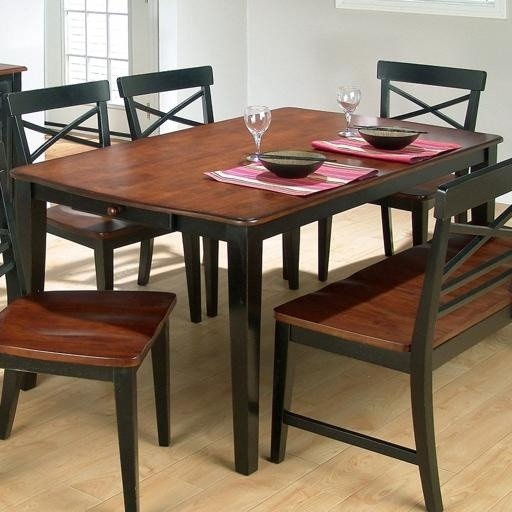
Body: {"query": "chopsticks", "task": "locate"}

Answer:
[252,153,339,165]
[345,123,429,135]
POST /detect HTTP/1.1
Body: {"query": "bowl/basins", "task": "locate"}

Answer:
[257,150,327,178]
[355,126,421,151]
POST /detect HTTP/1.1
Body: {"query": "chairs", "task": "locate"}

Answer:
[264,156,512,512]
[117,64,302,320]
[4,77,204,325]
[0,141,178,512]
[316,59,490,284]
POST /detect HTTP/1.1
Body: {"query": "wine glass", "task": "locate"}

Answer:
[337,86,362,138]
[243,105,271,162]
